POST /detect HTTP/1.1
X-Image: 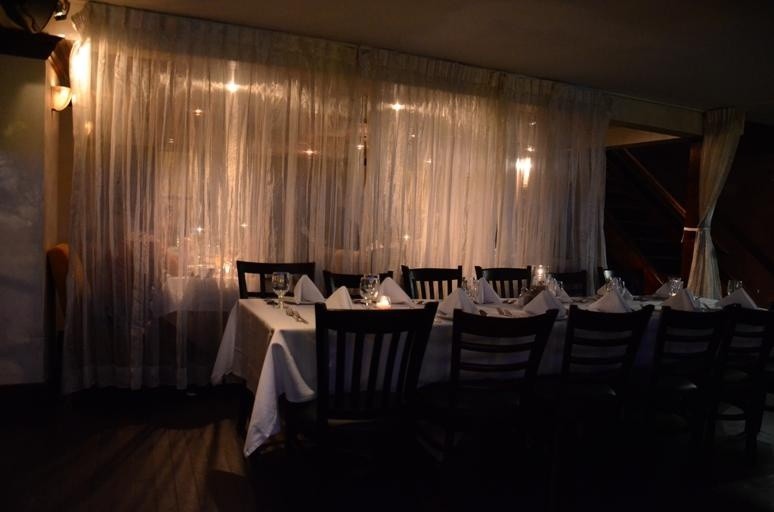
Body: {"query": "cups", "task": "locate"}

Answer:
[668,277,684,297]
[605,277,626,298]
[727,280,743,296]
[462,275,481,302]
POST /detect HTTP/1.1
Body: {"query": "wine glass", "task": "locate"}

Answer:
[359,276,380,310]
[271,271,292,312]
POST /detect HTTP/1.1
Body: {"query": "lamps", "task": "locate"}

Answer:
[50,85,71,112]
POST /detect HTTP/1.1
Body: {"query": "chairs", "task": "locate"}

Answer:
[550,269,589,298]
[401,264,463,300]
[48,243,148,381]
[322,268,394,300]
[646,302,744,393]
[281,299,439,436]
[237,261,317,298]
[707,301,772,470]
[474,265,532,298]
[427,307,562,407]
[557,303,654,389]
[597,265,646,296]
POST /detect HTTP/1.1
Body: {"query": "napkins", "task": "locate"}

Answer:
[324,286,352,310]
[596,277,634,300]
[661,288,701,312]
[558,286,574,303]
[587,288,627,313]
[656,277,684,298]
[714,288,758,310]
[472,277,502,305]
[293,275,325,304]
[439,287,479,317]
[524,290,568,319]
[375,276,411,302]
